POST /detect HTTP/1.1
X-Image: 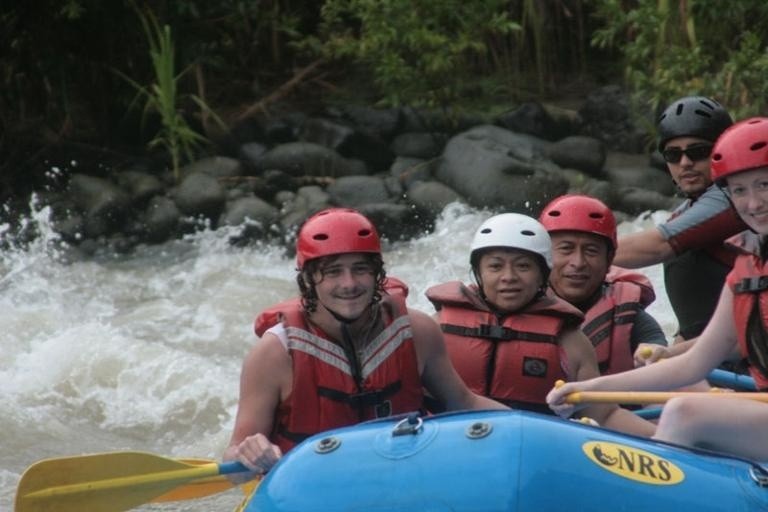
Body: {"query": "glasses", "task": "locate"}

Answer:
[662,139,712,164]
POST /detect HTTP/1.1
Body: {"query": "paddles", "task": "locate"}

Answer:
[14,452,251,511]
[149,461,263,501]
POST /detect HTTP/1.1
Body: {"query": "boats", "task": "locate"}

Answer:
[235,408,767,512]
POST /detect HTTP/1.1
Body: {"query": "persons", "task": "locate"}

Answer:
[634,335,743,369]
[425,212,658,438]
[538,195,667,411]
[546,116,768,463]
[611,95,752,375]
[219,207,512,494]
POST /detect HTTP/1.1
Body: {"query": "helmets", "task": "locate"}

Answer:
[470,211,554,274]
[654,95,733,152]
[296,207,380,270]
[710,114,767,186]
[539,193,619,268]
